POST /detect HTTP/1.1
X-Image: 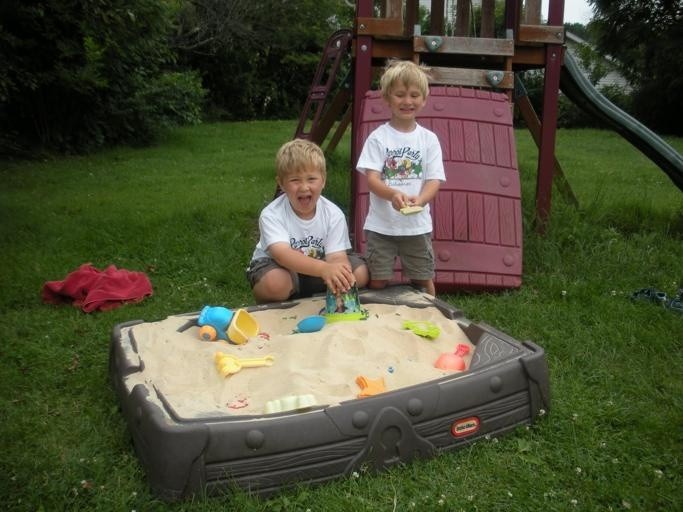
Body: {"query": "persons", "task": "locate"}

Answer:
[355,60,446,298]
[245,138,369,303]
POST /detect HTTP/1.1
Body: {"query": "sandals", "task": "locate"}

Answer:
[669,289,683,312]
[630,288,666,302]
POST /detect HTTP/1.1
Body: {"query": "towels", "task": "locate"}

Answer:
[38,260,154,316]
